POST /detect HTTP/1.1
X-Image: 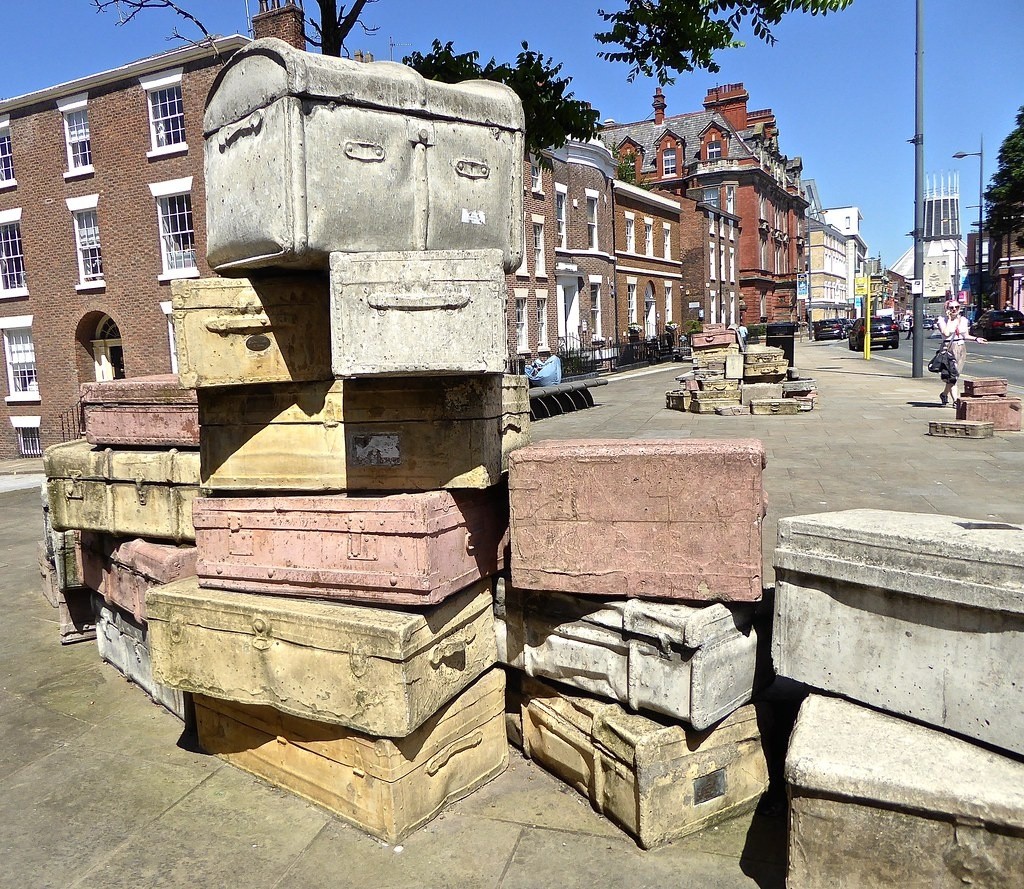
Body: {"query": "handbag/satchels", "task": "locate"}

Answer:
[928,350,944,373]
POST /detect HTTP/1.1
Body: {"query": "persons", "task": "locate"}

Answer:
[936,299,988,409]
[906,314,914,340]
[737,324,749,345]
[986,304,995,312]
[1003,298,1015,311]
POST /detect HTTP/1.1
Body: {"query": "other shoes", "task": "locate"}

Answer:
[952,401,957,409]
[939,392,948,405]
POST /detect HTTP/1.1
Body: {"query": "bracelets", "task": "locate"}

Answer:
[975,337,978,340]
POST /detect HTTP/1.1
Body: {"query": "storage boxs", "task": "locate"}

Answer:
[43,37,1024,889]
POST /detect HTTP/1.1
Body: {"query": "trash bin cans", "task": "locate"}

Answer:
[766,322,794,368]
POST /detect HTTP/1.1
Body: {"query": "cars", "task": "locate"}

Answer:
[897,313,940,331]
[836,318,855,339]
[977,309,1024,341]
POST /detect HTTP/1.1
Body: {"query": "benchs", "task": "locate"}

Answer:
[527,379,606,421]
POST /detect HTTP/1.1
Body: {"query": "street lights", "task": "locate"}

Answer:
[952,132,984,340]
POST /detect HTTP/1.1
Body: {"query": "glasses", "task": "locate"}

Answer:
[949,305,961,310]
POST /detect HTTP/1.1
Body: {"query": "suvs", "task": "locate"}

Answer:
[813,319,848,341]
[846,315,900,353]
